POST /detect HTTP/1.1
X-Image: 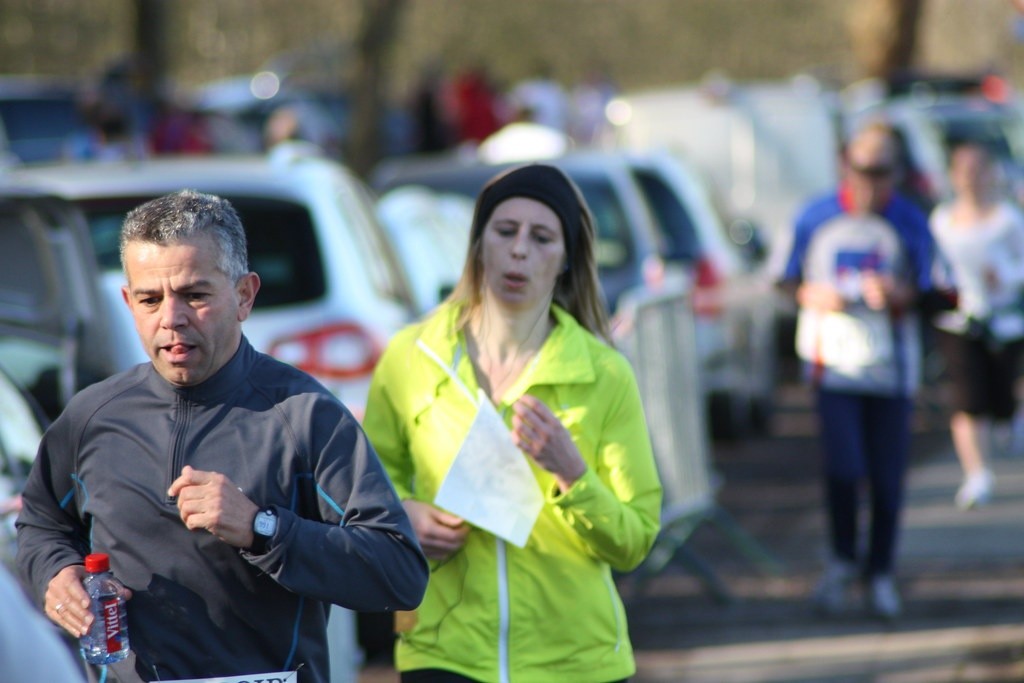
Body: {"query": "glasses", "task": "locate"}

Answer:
[849,163,904,181]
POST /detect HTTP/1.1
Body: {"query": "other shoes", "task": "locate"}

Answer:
[870,575,903,620]
[819,559,853,607]
[956,468,999,512]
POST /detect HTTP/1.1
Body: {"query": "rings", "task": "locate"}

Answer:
[55,604,62,614]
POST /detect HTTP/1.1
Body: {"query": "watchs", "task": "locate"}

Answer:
[253,504,278,556]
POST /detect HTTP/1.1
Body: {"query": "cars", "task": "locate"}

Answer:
[0,54,1024,683]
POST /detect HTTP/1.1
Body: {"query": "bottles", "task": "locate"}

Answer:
[78,554,130,664]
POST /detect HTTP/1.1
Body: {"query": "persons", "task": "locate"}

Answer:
[362,164,664,683]
[56,44,617,164]
[776,126,957,620]
[15,188,430,683]
[927,119,1024,512]
